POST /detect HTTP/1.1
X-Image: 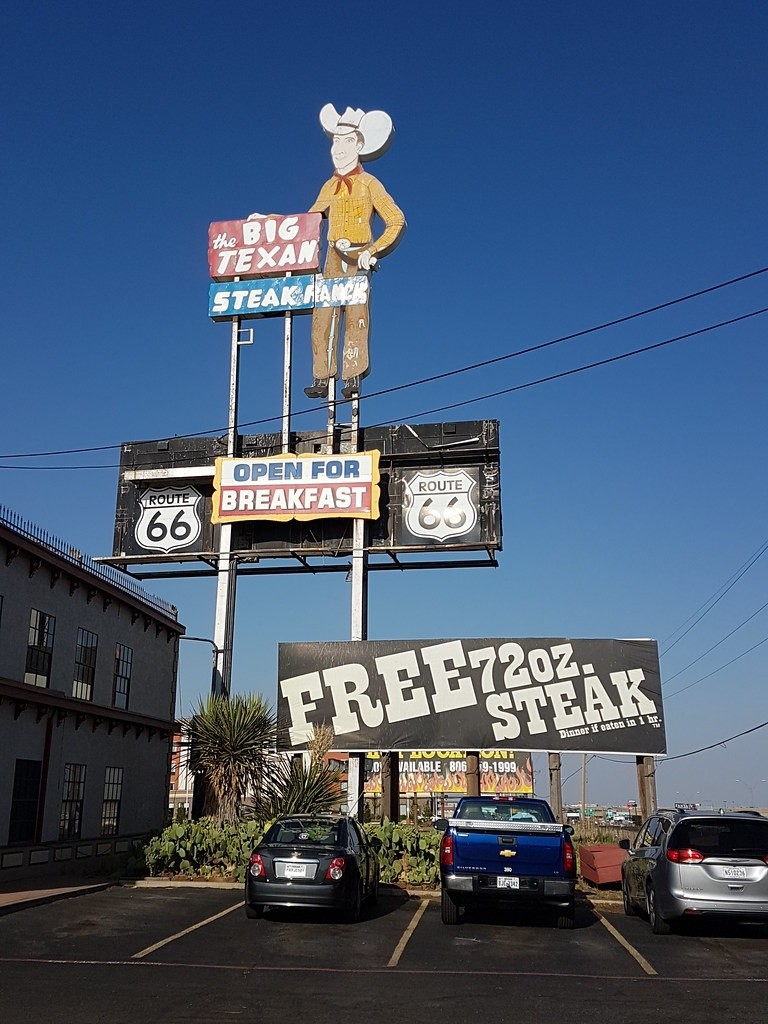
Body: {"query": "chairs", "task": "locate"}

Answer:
[321,833,335,843]
[281,831,295,842]
[467,806,484,818]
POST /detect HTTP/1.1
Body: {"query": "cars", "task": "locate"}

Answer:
[245,812,382,923]
[417,815,425,824]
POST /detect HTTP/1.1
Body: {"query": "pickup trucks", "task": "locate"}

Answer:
[432,795,577,929]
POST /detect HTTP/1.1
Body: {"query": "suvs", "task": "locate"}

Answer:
[618,808,768,937]
